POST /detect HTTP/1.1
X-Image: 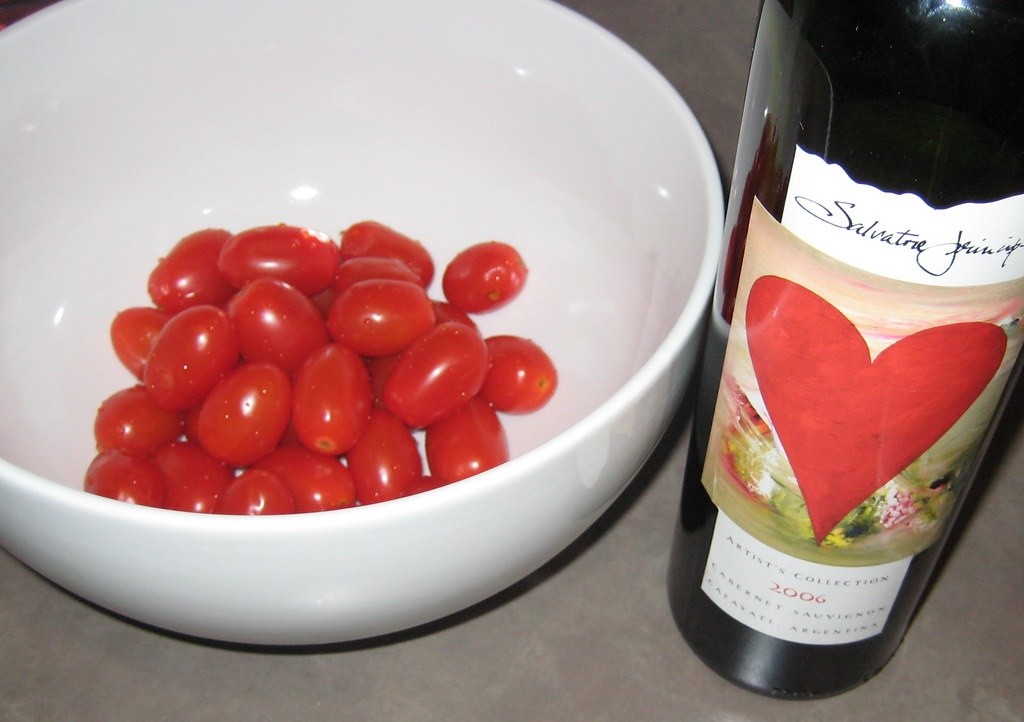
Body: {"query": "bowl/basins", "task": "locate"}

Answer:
[3,0,727,646]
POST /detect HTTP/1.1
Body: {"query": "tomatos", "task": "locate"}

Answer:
[83,222,559,510]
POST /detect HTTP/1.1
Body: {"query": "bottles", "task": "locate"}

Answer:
[666,0,1024,700]
